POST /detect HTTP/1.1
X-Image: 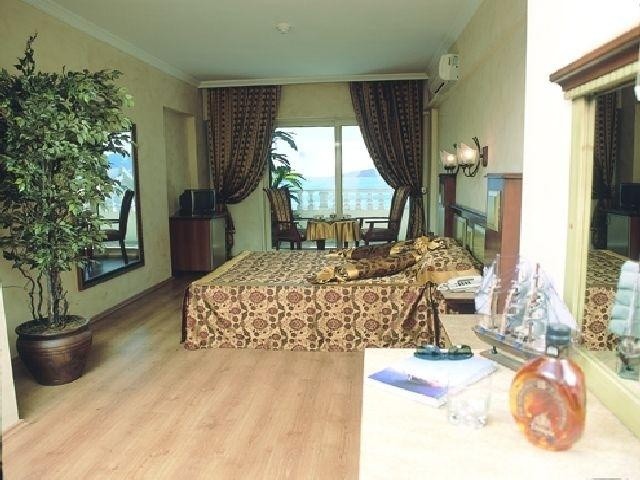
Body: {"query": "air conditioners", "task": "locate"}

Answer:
[428,53,460,96]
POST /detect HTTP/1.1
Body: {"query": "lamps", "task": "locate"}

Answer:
[456,137,488,177]
[439,143,458,176]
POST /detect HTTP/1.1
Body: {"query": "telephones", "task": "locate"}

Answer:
[447,275,482,293]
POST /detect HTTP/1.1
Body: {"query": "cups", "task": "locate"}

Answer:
[615,334,640,380]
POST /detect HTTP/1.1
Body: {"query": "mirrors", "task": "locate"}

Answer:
[550,26,640,438]
[71,125,145,292]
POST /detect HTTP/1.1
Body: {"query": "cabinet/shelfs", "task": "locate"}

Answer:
[170,214,231,276]
[598,207,640,261]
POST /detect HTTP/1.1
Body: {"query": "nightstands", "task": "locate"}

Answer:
[431,268,484,348]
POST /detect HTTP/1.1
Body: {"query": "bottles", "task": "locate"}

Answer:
[507,322,589,452]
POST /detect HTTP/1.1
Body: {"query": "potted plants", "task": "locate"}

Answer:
[0,30,138,387]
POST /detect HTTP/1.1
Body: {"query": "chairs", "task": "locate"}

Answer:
[355,183,413,248]
[82,189,135,277]
[263,186,306,249]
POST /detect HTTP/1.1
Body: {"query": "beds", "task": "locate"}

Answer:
[180,173,522,354]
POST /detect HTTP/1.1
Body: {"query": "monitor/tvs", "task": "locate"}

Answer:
[179,189,216,219]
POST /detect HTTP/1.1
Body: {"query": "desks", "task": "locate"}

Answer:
[361,314,640,480]
[306,220,360,249]
